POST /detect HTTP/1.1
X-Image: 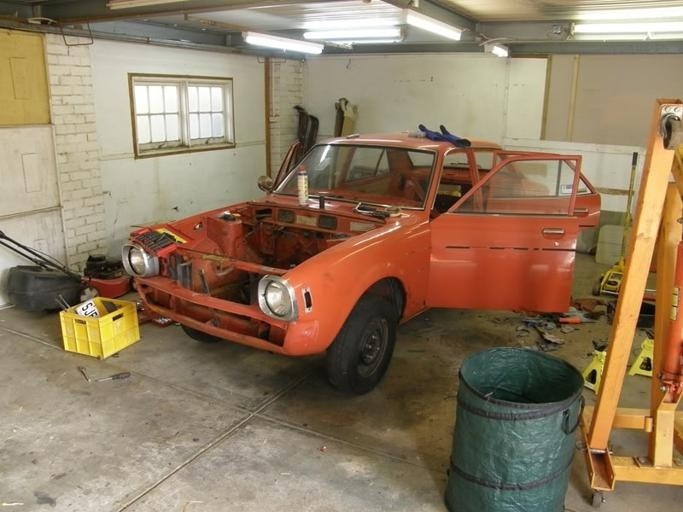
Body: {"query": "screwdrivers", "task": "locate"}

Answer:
[94,371,131,382]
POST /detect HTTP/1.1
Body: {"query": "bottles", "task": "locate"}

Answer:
[296,166,308,207]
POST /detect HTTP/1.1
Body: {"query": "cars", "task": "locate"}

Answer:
[121,133,602,397]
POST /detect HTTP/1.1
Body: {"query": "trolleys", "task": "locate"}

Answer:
[592,152,640,296]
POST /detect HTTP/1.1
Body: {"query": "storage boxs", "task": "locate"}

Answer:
[59,298,140,361]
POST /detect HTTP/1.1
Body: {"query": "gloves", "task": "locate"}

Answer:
[419,123,471,147]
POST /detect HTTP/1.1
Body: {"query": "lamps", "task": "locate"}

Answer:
[242,8,509,58]
[569,18,683,36]
